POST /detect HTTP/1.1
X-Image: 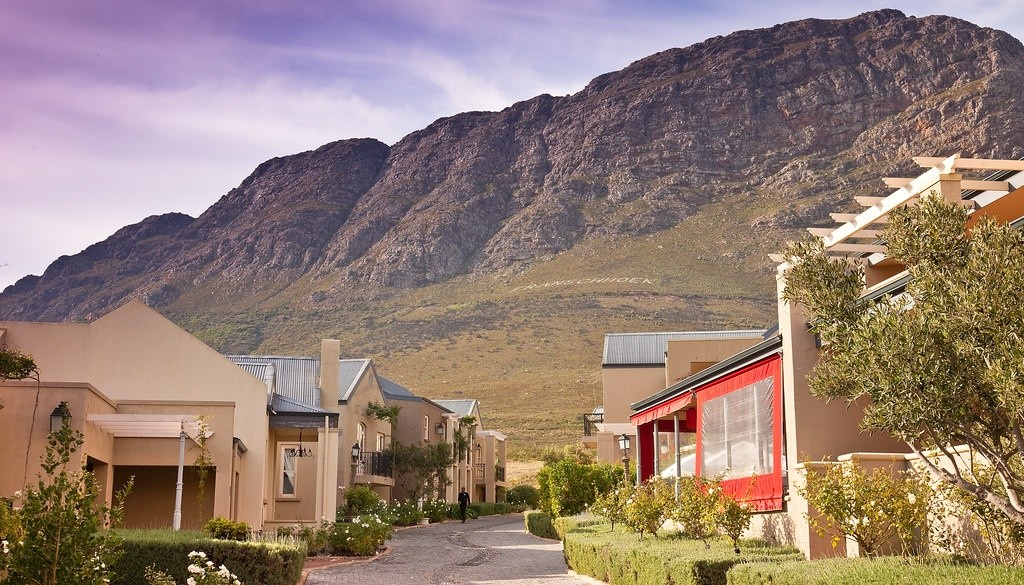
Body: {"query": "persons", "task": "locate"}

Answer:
[458,487,470,523]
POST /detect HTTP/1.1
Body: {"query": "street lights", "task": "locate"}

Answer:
[619,434,631,485]
[349,442,359,489]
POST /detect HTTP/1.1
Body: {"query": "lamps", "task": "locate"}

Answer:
[287,428,314,458]
[47,399,71,435]
[351,442,360,462]
[437,423,445,435]
[471,436,476,445]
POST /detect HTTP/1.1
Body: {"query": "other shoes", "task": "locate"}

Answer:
[462,520,465,523]
